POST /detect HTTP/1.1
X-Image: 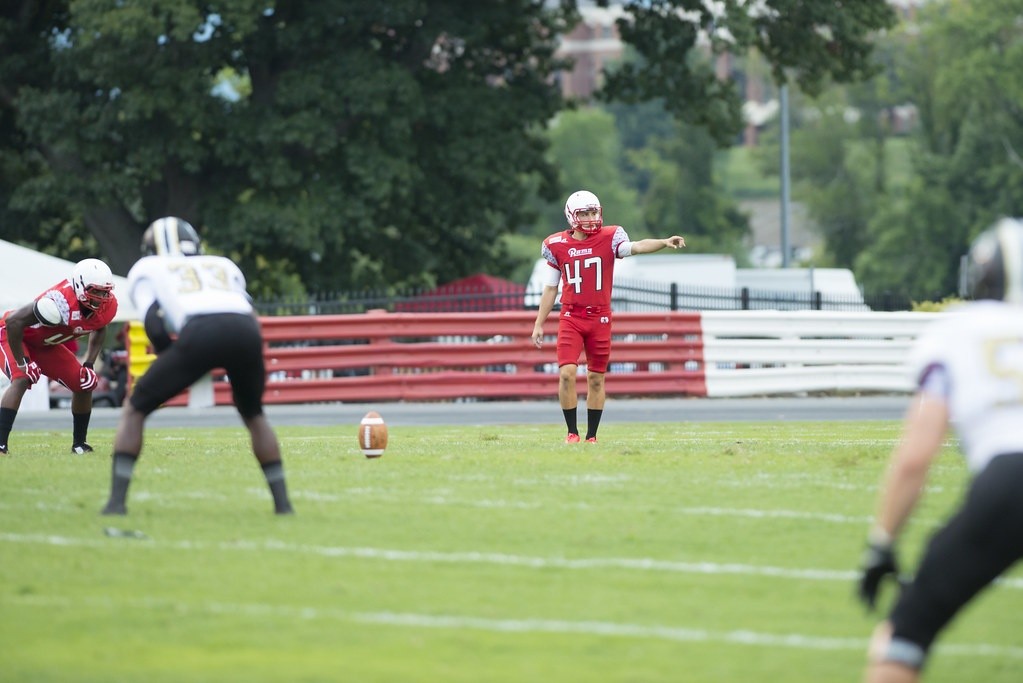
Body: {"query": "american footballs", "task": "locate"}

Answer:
[359,412,388,456]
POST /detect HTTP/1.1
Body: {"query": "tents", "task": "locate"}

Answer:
[0,239,139,409]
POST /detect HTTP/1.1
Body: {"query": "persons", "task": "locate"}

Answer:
[531,189,687,442]
[0,258,116,455]
[100,215,294,515]
[857,216,1023,683]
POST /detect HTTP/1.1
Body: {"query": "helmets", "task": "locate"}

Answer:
[959,216,1023,305]
[564,190,603,234]
[139,216,201,257]
[70,258,115,311]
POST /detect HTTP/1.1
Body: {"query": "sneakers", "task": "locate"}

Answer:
[566,433,580,443]
[584,435,596,443]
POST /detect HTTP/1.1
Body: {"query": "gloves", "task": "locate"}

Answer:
[856,541,898,610]
[17,356,42,384]
[80,362,99,392]
